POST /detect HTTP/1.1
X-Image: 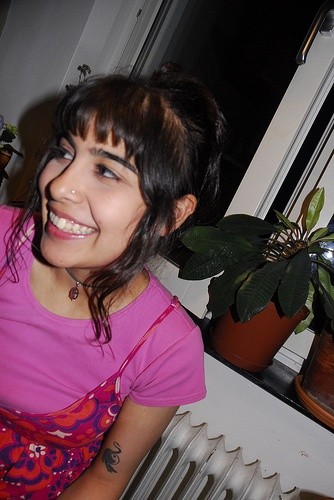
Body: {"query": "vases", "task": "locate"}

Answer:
[296,318,334,432]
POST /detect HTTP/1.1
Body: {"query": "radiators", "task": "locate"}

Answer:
[120,411,301,500]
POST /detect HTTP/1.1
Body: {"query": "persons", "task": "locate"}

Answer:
[1,64,228,500]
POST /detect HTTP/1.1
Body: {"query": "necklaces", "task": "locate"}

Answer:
[65,267,116,302]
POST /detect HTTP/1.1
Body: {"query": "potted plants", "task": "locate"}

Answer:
[181,186,334,374]
